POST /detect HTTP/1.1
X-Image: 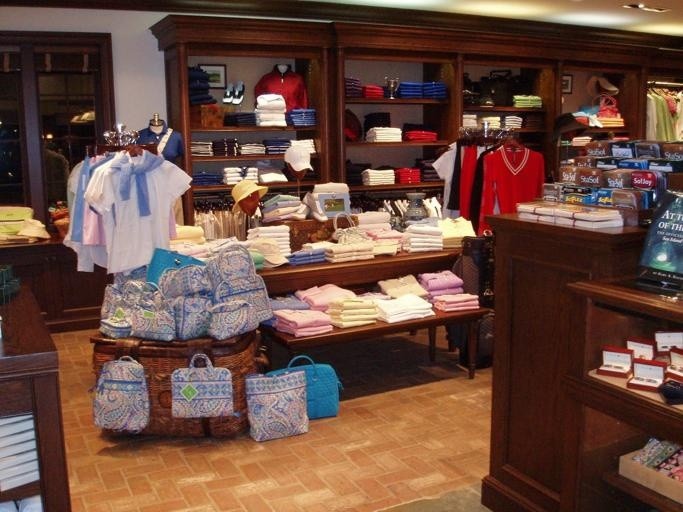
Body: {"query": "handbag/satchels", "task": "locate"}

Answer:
[599,105,617,117]
[246,371,309,442]
[98,245,274,341]
[481,69,517,106]
[333,227,372,245]
[267,356,339,420]
[92,355,149,433]
[172,353,234,418]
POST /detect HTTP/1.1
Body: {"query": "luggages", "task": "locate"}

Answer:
[444,236,495,367]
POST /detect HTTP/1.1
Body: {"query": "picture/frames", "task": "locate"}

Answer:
[560,74,574,95]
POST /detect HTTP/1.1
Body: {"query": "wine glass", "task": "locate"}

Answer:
[386,78,400,100]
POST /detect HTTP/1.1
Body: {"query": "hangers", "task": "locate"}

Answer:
[424,189,444,199]
[289,191,311,201]
[260,191,282,206]
[195,195,236,218]
[647,80,682,94]
[350,191,383,213]
[382,190,409,200]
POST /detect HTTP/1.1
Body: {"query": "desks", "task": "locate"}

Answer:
[235,238,490,380]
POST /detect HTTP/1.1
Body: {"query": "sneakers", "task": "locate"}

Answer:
[302,193,329,222]
[292,204,310,220]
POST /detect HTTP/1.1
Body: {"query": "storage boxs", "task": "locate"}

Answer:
[190,104,226,130]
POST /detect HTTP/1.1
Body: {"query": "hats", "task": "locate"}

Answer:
[284,145,314,171]
[17,219,52,239]
[587,77,619,97]
[250,239,290,269]
[230,180,268,213]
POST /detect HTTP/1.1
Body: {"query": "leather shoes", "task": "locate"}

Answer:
[223,81,245,105]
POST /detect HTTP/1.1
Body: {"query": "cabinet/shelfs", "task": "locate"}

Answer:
[520,29,647,184]
[479,212,681,510]
[423,26,555,184]
[334,19,463,199]
[616,34,683,139]
[0,280,72,512]
[148,14,338,225]
